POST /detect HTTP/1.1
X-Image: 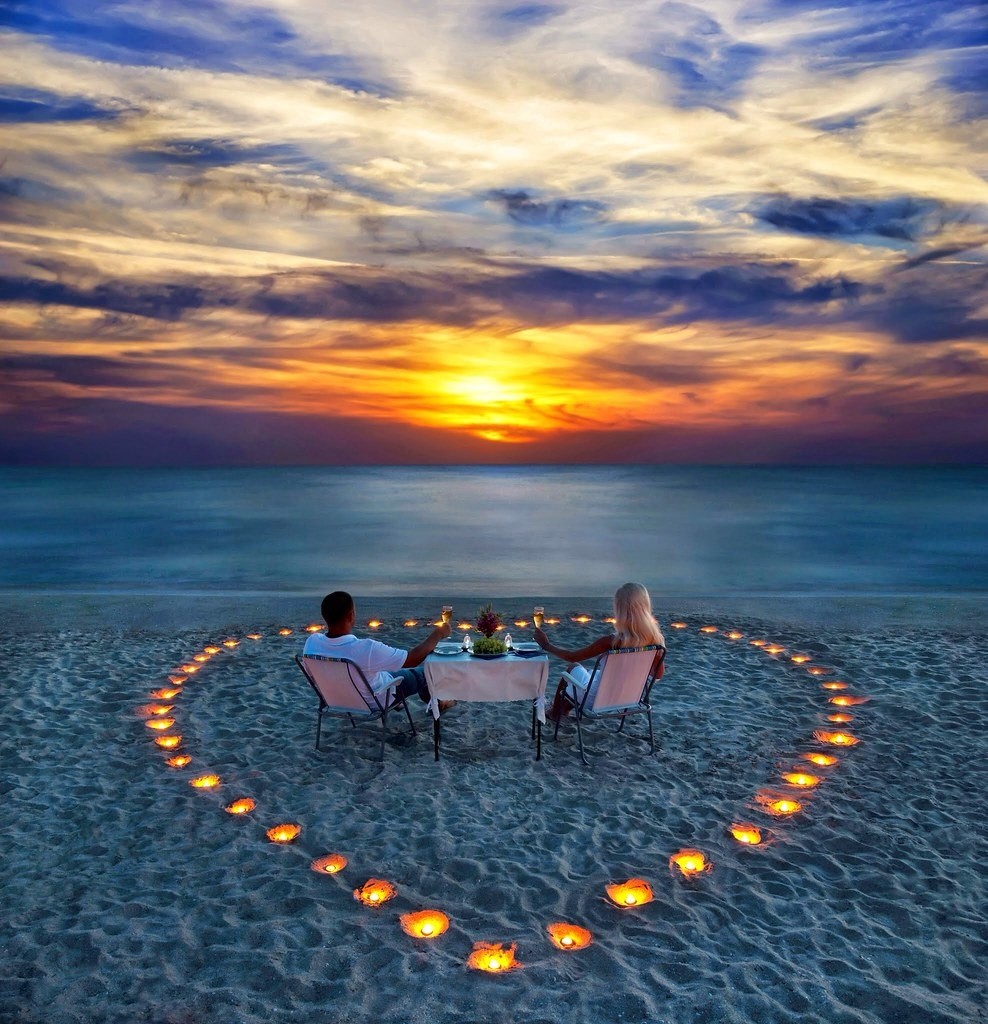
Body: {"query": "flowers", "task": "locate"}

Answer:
[474,601,502,637]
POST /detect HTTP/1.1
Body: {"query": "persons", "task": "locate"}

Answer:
[532,581,667,723]
[301,591,458,718]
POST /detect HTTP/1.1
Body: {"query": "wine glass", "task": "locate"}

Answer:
[442,605,453,640]
[533,607,545,640]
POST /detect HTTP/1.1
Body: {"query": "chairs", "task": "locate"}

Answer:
[554,645,666,765]
[295,653,417,762]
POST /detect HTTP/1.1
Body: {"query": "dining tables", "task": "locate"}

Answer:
[425,640,550,761]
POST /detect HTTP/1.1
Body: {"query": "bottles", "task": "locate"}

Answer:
[505,633,512,650]
[463,633,470,649]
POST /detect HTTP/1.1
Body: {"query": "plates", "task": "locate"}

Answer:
[513,643,543,654]
[434,646,463,655]
